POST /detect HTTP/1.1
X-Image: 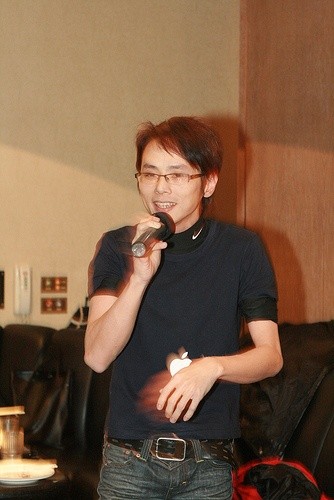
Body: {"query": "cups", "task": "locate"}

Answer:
[0,413,25,460]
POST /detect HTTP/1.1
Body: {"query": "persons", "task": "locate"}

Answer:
[84,116,282,500]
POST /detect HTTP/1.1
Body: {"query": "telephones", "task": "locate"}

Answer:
[13,264,32,317]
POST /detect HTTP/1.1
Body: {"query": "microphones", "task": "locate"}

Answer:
[132,211,176,258]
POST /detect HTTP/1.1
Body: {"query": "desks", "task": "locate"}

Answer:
[0,470,71,500]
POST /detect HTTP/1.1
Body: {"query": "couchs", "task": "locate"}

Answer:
[0,319,334,500]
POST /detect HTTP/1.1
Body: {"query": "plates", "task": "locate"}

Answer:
[1,456,58,487]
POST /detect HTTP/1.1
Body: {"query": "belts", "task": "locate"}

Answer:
[103,434,238,467]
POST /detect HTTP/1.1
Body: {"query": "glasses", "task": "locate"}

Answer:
[135,173,206,184]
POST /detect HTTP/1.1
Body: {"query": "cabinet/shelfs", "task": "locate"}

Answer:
[237,0,334,337]
